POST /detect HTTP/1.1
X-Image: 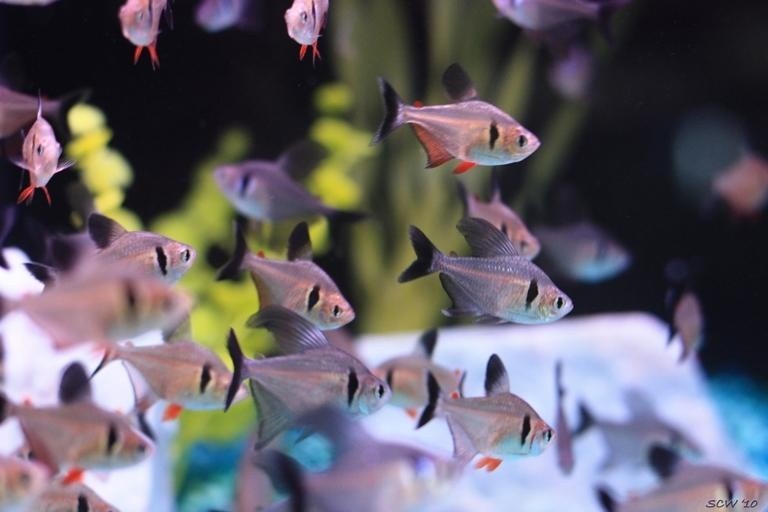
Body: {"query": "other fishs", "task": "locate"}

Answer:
[2,1,768,512]
